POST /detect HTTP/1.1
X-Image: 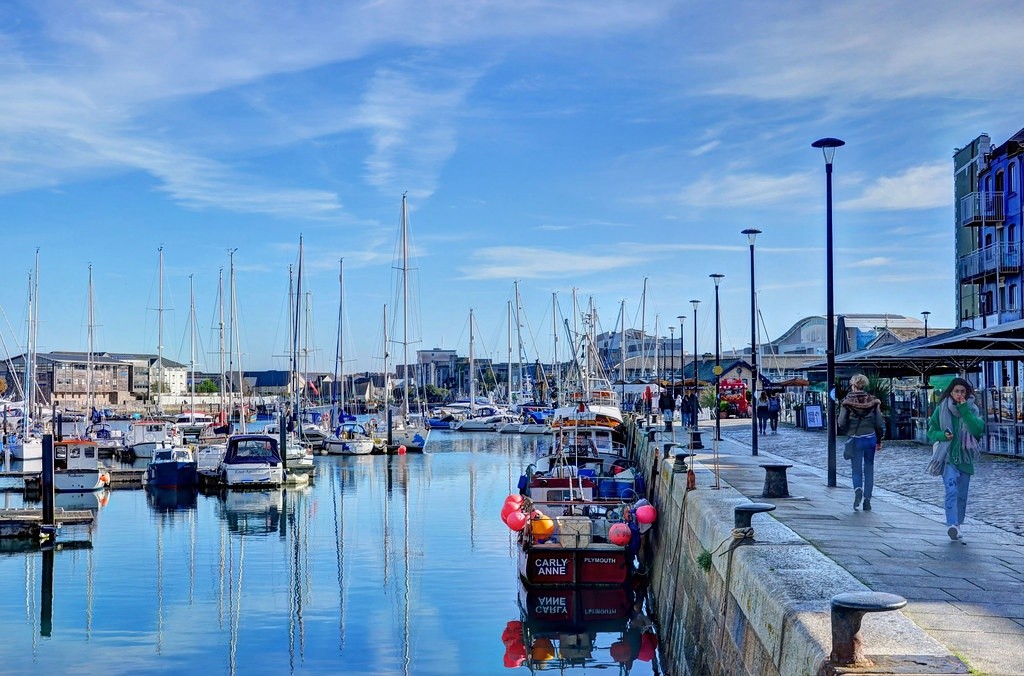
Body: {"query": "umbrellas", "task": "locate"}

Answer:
[807,327,980,383]
[834,315,850,356]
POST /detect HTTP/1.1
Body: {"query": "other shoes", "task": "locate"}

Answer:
[854,495,861,511]
[948,527,958,541]
[958,530,963,538]
[863,503,872,510]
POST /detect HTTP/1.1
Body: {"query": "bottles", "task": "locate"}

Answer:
[635,474,645,493]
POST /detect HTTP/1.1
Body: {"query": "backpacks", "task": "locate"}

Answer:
[770,397,778,412]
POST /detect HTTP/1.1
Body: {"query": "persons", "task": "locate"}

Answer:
[91,407,98,425]
[757,391,780,434]
[659,390,704,429]
[838,374,884,511]
[926,378,984,540]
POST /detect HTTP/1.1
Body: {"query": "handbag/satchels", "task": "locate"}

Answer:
[844,438,855,460]
[929,441,942,476]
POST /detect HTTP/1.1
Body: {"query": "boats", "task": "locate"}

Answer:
[515,569,644,676]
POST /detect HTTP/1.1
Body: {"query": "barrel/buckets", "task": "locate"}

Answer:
[616,480,634,498]
[598,479,615,497]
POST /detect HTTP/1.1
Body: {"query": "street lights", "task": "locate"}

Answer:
[812,137,847,487]
[741,228,763,453]
[677,316,687,399]
[668,326,676,399]
[689,299,701,431]
[661,336,668,380]
[708,273,725,441]
[921,311,931,337]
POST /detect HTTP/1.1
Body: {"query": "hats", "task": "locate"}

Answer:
[661,389,665,392]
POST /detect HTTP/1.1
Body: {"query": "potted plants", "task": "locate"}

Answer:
[720,401,729,419]
[834,375,848,436]
[863,370,892,440]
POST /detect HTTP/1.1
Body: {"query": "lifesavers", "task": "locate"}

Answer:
[129,425,133,431]
[342,432,347,438]
[322,414,329,422]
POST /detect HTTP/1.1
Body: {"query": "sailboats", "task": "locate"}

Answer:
[0,189,657,592]
